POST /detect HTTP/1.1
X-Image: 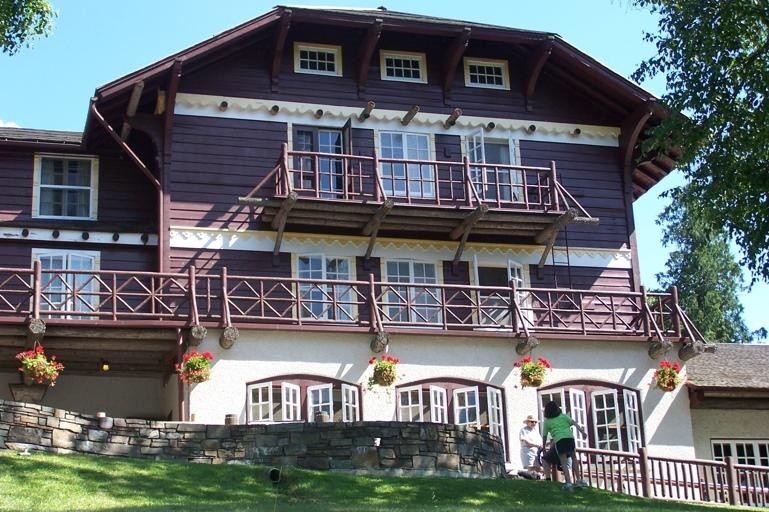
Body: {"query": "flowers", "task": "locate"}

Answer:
[171,352,214,384]
[652,359,684,390]
[513,356,551,390]
[15,346,65,389]
[366,355,405,396]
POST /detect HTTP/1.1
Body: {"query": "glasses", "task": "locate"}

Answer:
[531,421,537,423]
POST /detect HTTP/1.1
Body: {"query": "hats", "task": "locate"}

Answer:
[523,415,537,424]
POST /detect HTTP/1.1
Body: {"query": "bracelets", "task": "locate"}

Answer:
[580,429,584,433]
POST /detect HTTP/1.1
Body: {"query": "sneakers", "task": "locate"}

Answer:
[562,483,572,490]
[577,480,584,487]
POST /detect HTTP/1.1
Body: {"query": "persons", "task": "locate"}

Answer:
[542,401,587,490]
[542,441,572,481]
[519,414,544,477]
